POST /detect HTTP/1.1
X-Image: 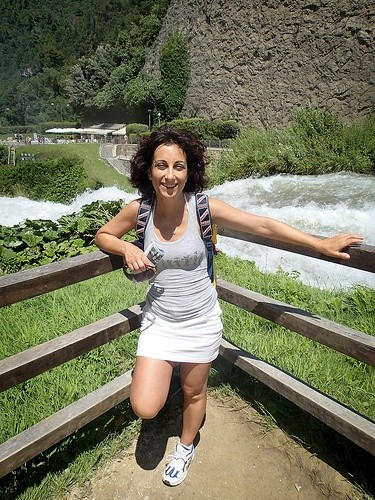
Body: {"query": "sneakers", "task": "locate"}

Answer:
[162,443,195,486]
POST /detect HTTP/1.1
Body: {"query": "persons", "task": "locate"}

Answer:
[95,129,363,486]
[14,131,44,145]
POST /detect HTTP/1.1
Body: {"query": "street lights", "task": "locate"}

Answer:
[145,109,153,131]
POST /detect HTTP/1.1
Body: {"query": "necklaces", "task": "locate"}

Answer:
[159,207,184,235]
